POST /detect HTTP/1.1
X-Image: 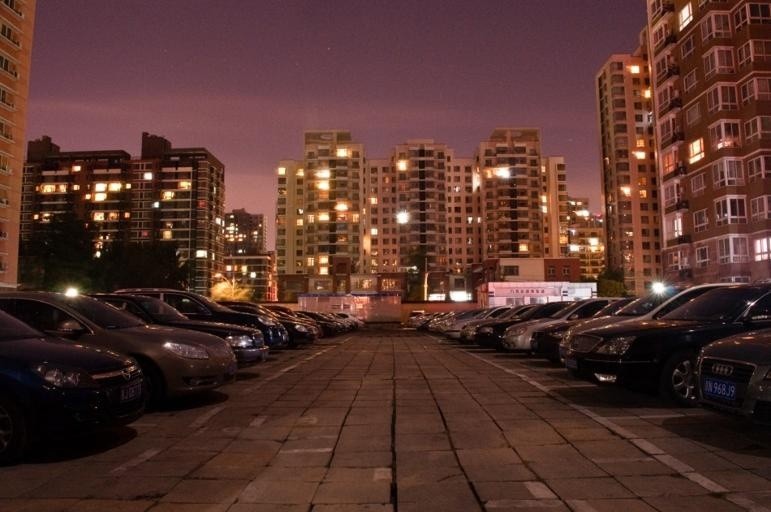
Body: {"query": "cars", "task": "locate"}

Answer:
[693,328,771,418]
[408,296,639,365]
[0,291,239,406]
[0,308,144,464]
[84,286,366,371]
[558,280,771,407]
